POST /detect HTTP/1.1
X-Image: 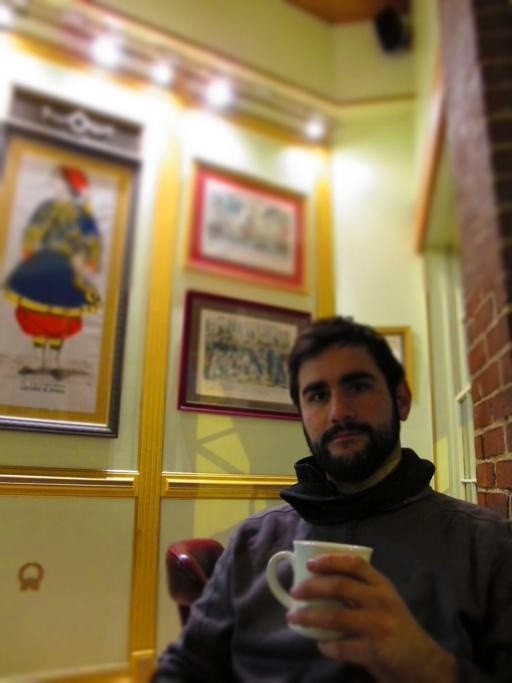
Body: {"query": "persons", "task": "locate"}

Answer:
[147,316,512,682]
[2,163,101,381]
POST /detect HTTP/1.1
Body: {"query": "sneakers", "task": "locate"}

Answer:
[19,362,47,376]
[50,367,64,380]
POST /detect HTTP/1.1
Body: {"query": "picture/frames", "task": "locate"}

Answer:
[176,288,313,423]
[179,155,316,297]
[0,114,147,440]
[378,324,413,393]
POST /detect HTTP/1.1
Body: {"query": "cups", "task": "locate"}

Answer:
[263,541,381,643]
